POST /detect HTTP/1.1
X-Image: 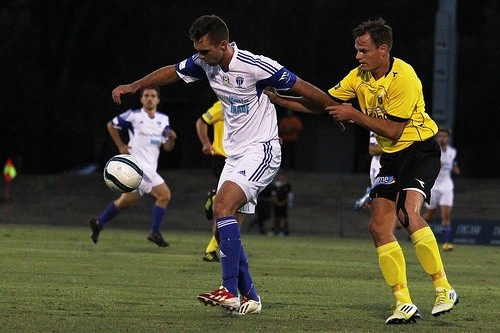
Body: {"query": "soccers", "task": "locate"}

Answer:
[103,154,144,194]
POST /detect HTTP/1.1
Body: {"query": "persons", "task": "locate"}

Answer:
[278,108,305,171]
[88,86,177,247]
[271,171,295,236]
[113,17,355,316]
[196,101,227,262]
[246,183,274,234]
[353,131,380,211]
[420,126,460,252]
[263,17,458,325]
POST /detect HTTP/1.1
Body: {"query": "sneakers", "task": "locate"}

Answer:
[196,286,262,317]
[442,241,456,255]
[385,305,420,323]
[147,227,170,248]
[89,217,101,245]
[204,251,220,263]
[429,286,459,317]
